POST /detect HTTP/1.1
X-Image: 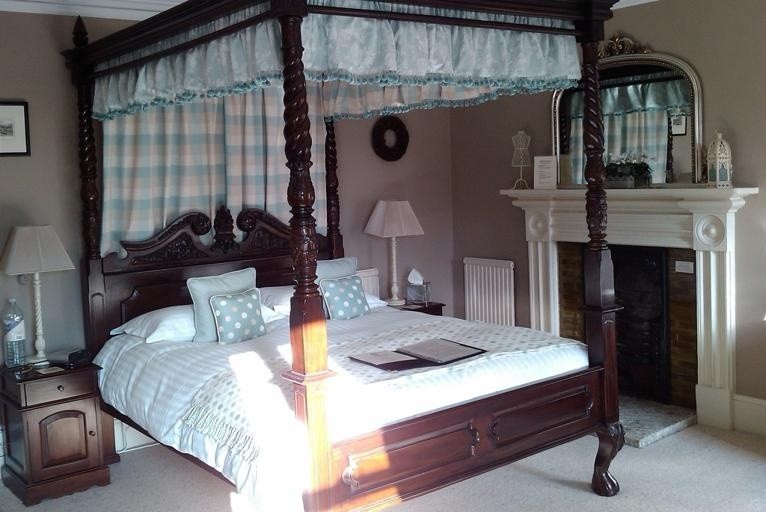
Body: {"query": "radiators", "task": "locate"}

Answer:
[462,256,515,328]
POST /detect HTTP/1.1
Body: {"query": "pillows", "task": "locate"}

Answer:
[316,258,358,298]
[209,288,266,344]
[268,293,388,317]
[109,302,286,344]
[319,274,371,320]
[187,268,256,342]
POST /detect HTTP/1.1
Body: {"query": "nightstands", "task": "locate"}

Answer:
[399,302,446,316]
[0,357,111,507]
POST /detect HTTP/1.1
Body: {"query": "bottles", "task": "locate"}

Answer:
[2,298,27,369]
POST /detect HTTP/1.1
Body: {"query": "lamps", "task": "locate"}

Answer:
[362,199,424,307]
[1,225,76,371]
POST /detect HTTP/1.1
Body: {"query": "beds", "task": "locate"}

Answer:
[60,1,626,512]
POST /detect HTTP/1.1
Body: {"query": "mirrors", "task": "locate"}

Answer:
[551,33,704,189]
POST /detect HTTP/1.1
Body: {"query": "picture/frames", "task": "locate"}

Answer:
[671,114,688,136]
[0,102,31,157]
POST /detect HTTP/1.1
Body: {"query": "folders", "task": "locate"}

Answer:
[345,337,489,373]
